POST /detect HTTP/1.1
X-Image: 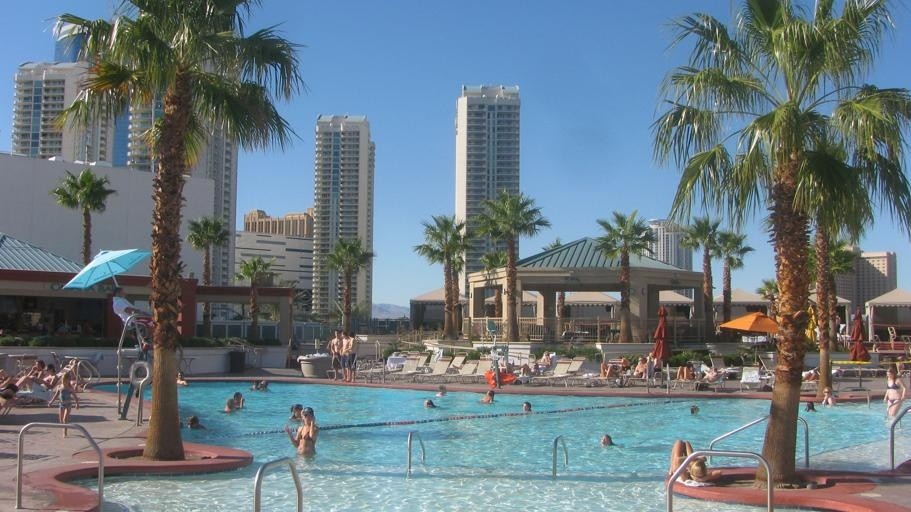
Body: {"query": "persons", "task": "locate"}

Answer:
[668,437,723,482]
[676,358,725,414]
[479,390,495,406]
[522,401,531,411]
[436,386,445,398]
[600,435,616,447]
[424,399,435,408]
[0,360,80,423]
[802,364,837,412]
[225,376,269,414]
[325,329,358,384]
[187,415,208,429]
[508,349,554,378]
[176,371,190,386]
[111,286,155,328]
[882,352,907,428]
[284,403,320,456]
[600,350,663,387]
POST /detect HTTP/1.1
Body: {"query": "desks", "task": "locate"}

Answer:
[124,354,198,377]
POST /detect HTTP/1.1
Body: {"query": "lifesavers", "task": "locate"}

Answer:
[130,361,152,387]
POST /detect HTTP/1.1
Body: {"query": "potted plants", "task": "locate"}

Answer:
[703,331,743,358]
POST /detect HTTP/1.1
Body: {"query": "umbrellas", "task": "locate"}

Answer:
[650,304,670,388]
[719,311,780,362]
[61,246,152,291]
[849,306,871,386]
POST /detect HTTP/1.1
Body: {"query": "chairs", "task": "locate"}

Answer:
[325,349,841,390]
[1,350,105,409]
